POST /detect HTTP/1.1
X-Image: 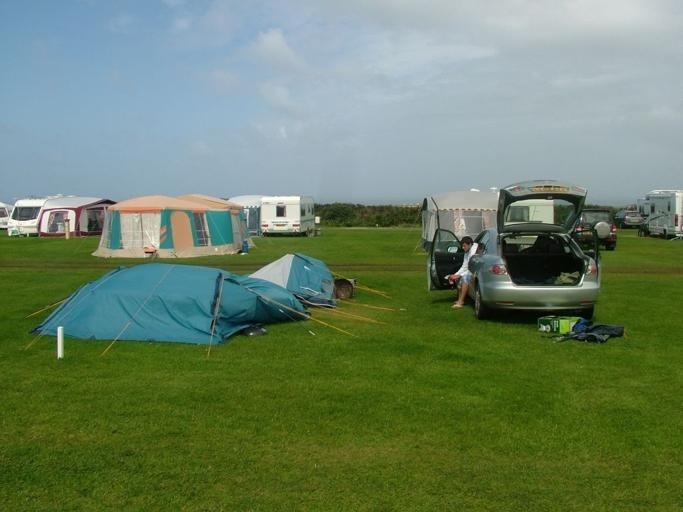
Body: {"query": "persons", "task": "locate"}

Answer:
[447,236,478,309]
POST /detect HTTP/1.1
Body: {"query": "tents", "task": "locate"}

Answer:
[505,198,555,225]
[37,197,117,237]
[90,195,255,258]
[248,252,336,306]
[228,194,269,237]
[420,193,555,256]
[29,263,310,346]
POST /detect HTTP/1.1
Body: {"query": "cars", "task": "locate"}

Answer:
[426,179,601,321]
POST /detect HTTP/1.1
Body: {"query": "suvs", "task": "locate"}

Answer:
[613,210,645,230]
[572,208,617,251]
